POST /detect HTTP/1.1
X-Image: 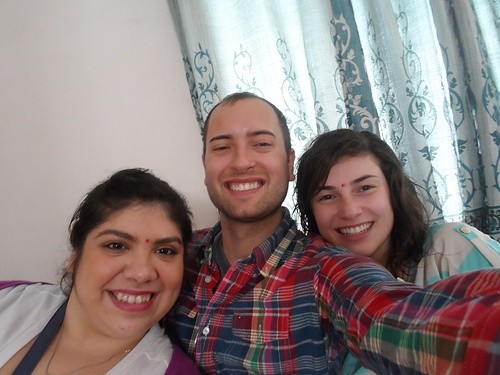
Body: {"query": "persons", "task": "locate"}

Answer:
[291,128,500,375]
[158,92,499,375]
[0,168,199,375]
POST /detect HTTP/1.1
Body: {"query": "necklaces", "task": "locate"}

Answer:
[46,327,132,375]
